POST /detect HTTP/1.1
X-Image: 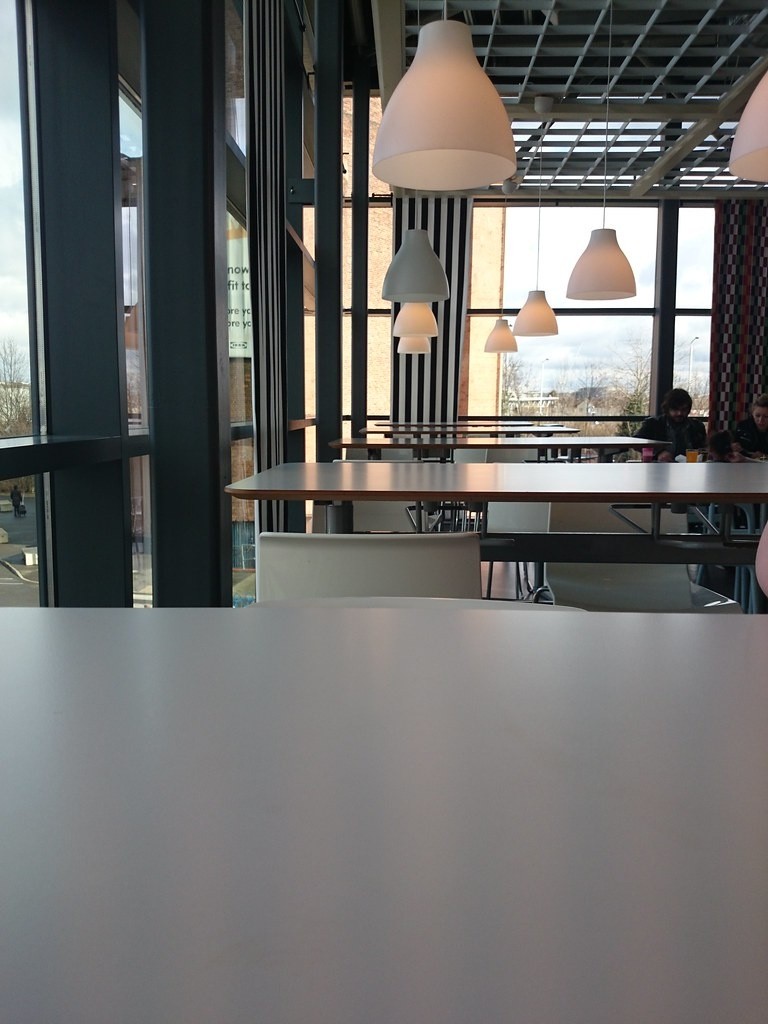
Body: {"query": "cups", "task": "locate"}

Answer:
[685,449,698,463]
[641,448,654,463]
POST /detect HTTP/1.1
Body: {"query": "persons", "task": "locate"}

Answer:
[10,485,22,518]
[632,389,706,462]
[706,393,768,463]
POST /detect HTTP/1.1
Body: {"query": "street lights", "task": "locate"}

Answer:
[539,357,550,415]
[686,336,701,396]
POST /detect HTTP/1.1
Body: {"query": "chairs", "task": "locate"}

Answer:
[251,523,486,606]
[534,498,751,613]
[486,502,550,599]
[333,500,443,533]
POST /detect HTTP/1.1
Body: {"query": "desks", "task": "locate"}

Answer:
[374,418,536,428]
[0,605,768,1024]
[222,461,768,615]
[357,424,583,437]
[328,434,673,462]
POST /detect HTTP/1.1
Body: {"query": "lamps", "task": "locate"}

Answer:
[392,304,439,339]
[382,190,454,303]
[372,0,519,190]
[565,0,639,302]
[729,72,768,183]
[484,314,518,355]
[396,335,432,356]
[510,126,559,338]
